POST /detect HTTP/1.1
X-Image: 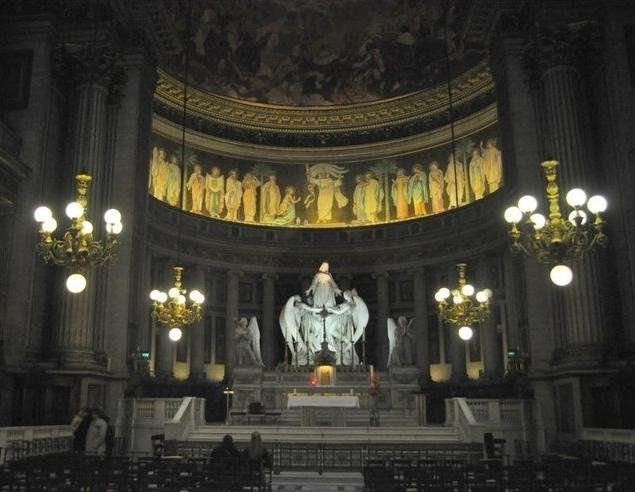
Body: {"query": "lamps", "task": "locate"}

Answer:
[32,174,123,295]
[149,1,205,344]
[434,1,494,343]
[503,158,608,287]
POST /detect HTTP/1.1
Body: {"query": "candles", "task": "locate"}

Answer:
[285,326,366,342]
[350,387,353,395]
[293,388,296,397]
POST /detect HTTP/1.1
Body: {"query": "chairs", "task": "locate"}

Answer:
[360,445,635,492]
[0,444,274,492]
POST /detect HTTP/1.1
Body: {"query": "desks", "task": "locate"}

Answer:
[287,395,360,427]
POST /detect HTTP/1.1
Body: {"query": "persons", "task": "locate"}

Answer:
[176,4,436,109]
[234,316,261,368]
[328,287,355,356]
[207,431,242,485]
[291,295,325,352]
[305,261,342,312]
[71,408,92,452]
[83,408,107,453]
[240,431,275,488]
[391,314,414,364]
